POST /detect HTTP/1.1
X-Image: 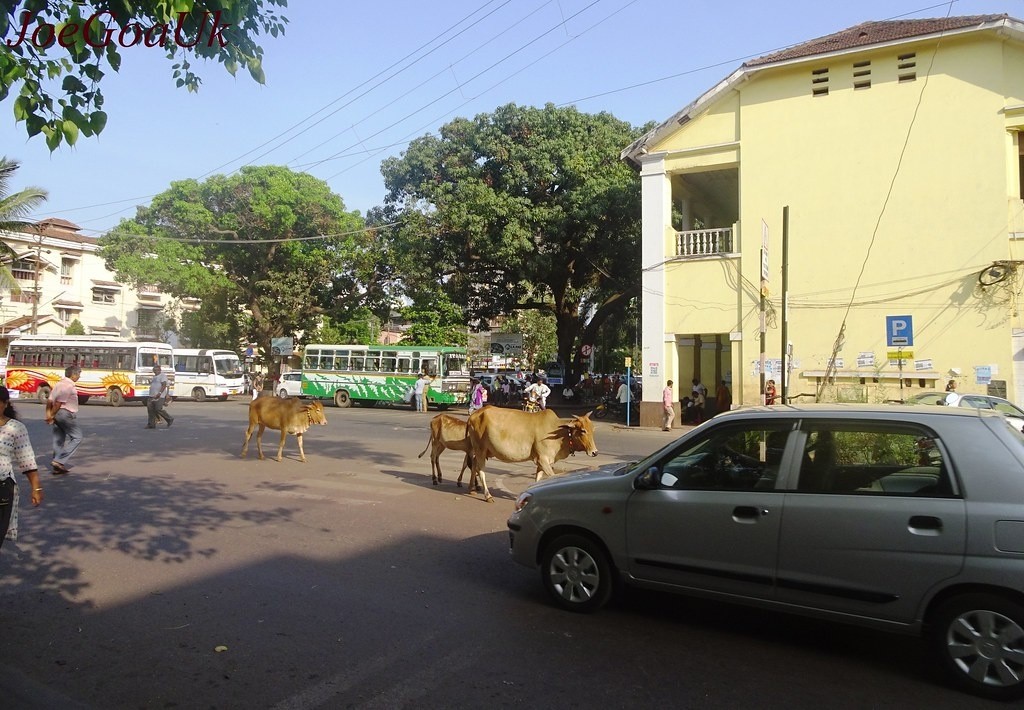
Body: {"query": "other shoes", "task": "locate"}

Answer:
[167,417,174,426]
[145,424,156,429]
[663,428,672,432]
[51,469,61,475]
[51,460,69,472]
[156,420,163,424]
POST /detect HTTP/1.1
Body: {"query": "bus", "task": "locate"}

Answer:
[174,349,244,402]
[300,344,471,411]
[5,335,176,407]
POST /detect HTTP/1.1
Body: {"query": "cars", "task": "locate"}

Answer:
[907,392,1024,433]
[507,403,1024,699]
[276,372,323,401]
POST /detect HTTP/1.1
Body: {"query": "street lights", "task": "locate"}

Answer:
[28,222,51,334]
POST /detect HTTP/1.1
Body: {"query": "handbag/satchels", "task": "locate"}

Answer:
[142,398,148,406]
[482,389,487,402]
[257,386,262,391]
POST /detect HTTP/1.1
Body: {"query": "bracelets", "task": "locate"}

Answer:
[35,488,43,491]
[159,391,161,394]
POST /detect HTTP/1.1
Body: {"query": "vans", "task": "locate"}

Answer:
[474,372,529,396]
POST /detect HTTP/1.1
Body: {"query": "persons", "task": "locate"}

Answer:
[44,366,84,474]
[766,380,776,405]
[615,380,634,421]
[468,371,485,415]
[681,379,707,422]
[519,373,538,390]
[715,380,731,412]
[562,385,574,397]
[946,380,957,392]
[252,372,262,400]
[720,432,788,489]
[144,365,174,428]
[494,375,517,405]
[524,378,551,410]
[662,380,675,431]
[586,374,608,384]
[415,371,435,412]
[626,374,636,384]
[0,385,44,550]
[272,372,280,384]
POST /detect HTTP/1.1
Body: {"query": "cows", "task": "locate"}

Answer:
[418,413,494,488]
[466,405,600,504]
[242,396,327,463]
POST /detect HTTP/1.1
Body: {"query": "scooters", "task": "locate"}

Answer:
[593,390,636,419]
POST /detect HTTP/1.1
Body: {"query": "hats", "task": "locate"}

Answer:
[538,376,543,381]
[620,376,626,382]
[498,375,503,378]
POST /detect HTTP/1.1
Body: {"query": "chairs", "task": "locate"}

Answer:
[310,361,392,372]
[10,354,99,368]
[754,432,838,494]
